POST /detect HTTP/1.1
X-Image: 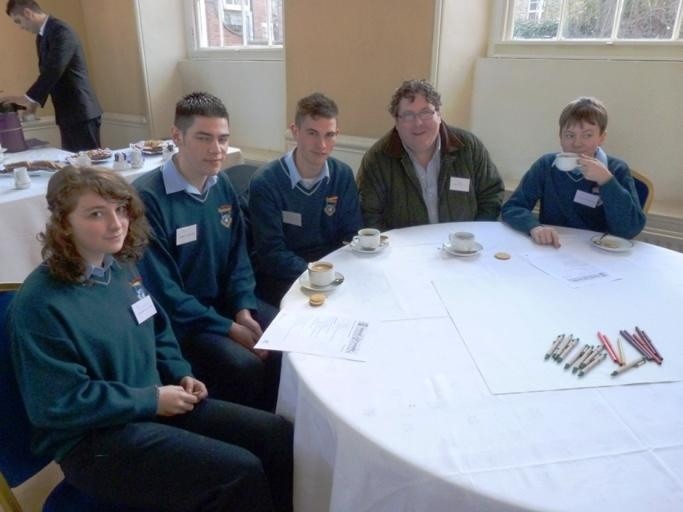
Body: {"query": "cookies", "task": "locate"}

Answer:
[495,253,511,260]
[310,294,325,306]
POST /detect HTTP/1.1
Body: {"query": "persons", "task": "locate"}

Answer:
[499,95,647,251]
[128,91,283,421]
[354,78,505,239]
[3,163,294,511]
[0,0,103,153]
[246,91,367,305]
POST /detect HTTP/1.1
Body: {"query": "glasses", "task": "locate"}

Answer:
[396,109,438,121]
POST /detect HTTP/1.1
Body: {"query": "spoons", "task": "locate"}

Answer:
[592,232,610,244]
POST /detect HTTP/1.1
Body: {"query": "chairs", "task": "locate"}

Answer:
[1,102,48,151]
[628,168,653,214]
[224,163,259,188]
[0,285,102,511]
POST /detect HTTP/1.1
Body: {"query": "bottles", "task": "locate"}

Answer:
[14,167,32,190]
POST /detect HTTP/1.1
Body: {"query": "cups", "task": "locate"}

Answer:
[351,228,380,248]
[555,152,584,171]
[449,232,477,252]
[307,260,337,286]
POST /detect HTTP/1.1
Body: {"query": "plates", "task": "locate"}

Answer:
[68,139,179,171]
[441,242,485,257]
[0,160,68,177]
[299,271,345,291]
[591,234,634,251]
[350,240,391,254]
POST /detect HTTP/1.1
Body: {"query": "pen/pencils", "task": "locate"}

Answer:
[544,332,606,377]
[598,327,663,375]
